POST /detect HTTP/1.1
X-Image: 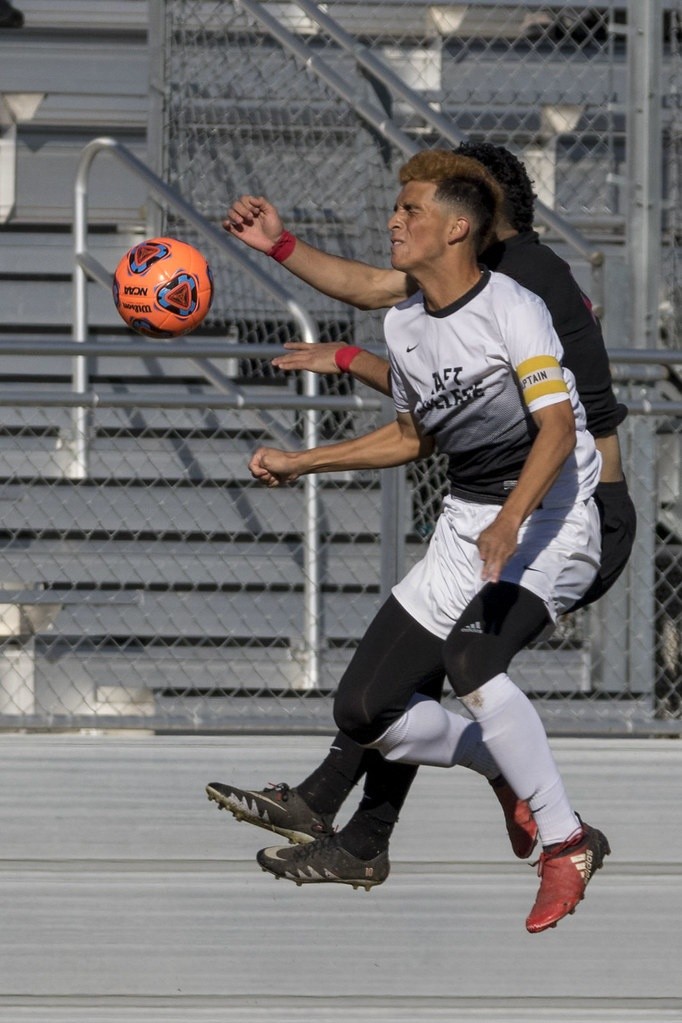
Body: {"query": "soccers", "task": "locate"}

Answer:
[111,237,217,340]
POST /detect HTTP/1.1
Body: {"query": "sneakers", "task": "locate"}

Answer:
[205,781,336,844]
[256,831,390,891]
[488,773,539,859]
[526,811,611,934]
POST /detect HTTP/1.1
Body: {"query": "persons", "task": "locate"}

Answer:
[248,145,610,936]
[207,138,635,888]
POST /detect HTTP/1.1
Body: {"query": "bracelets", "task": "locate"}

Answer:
[266,229,297,263]
[334,344,363,373]
